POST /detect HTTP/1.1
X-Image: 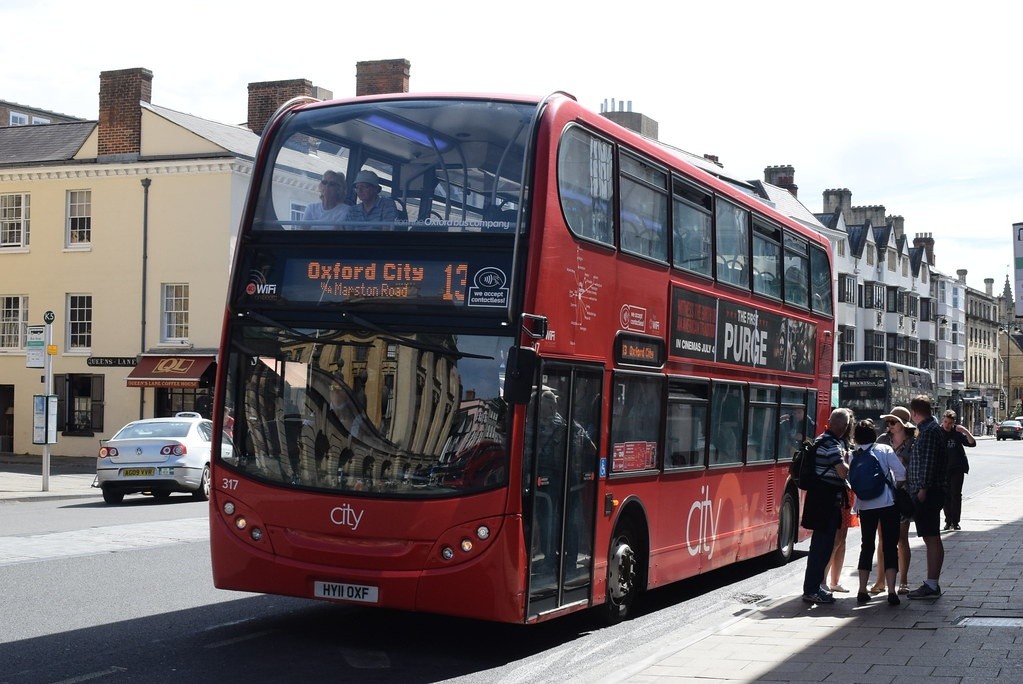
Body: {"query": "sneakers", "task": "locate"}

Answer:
[907,581,941,599]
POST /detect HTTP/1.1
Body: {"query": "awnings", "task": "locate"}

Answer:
[127,353,216,388]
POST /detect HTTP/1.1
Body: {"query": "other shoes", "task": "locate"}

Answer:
[831,584,850,592]
[820,584,831,593]
[944,523,951,530]
[866,583,886,593]
[888,593,900,606]
[953,524,961,530]
[898,584,909,594]
[802,592,836,605]
[857,592,871,604]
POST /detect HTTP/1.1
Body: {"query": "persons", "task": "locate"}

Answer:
[802,408,854,605]
[535,392,597,575]
[907,394,947,599]
[331,170,397,231]
[869,406,920,594]
[780,408,815,458]
[299,170,352,230]
[986,415,991,436]
[991,418,999,437]
[820,408,856,593]
[786,265,807,307]
[848,420,907,604]
[939,410,976,530]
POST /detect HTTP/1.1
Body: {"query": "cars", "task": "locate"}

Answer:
[996,421,1023,441]
[97,417,234,504]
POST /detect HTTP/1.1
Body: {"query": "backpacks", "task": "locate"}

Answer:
[788,436,836,491]
[849,444,892,500]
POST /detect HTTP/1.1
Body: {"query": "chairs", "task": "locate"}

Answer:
[389,197,824,311]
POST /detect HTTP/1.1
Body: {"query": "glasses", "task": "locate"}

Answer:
[321,179,340,187]
[885,419,899,425]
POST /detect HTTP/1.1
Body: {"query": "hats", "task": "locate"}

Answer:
[880,407,916,428]
[353,170,381,193]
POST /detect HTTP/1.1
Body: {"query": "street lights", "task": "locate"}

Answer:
[998,310,1023,420]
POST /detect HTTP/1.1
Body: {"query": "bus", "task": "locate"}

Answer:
[209,91,835,626]
[839,361,936,439]
